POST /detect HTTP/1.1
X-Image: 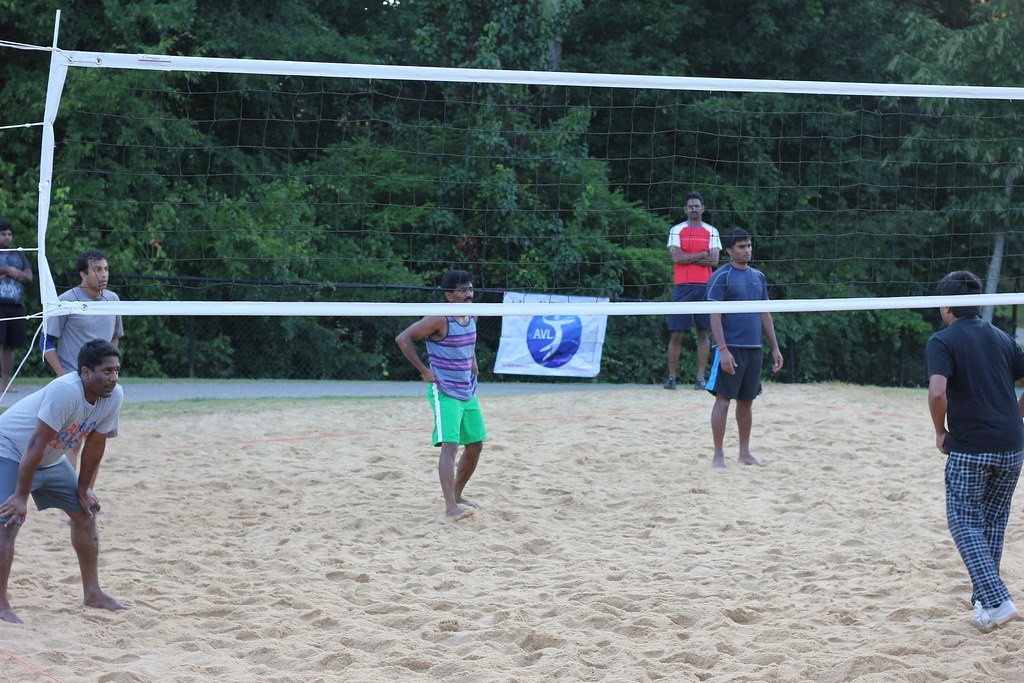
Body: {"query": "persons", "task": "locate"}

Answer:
[39,249,124,527]
[0,221,33,393]
[663,191,722,390]
[924,271,1024,632]
[703,228,783,467]
[0,338,124,624]
[395,269,489,517]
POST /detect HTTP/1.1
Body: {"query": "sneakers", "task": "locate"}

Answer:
[979,600,1020,629]
[974,601,983,621]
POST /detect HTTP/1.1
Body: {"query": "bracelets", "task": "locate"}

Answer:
[719,346,728,351]
